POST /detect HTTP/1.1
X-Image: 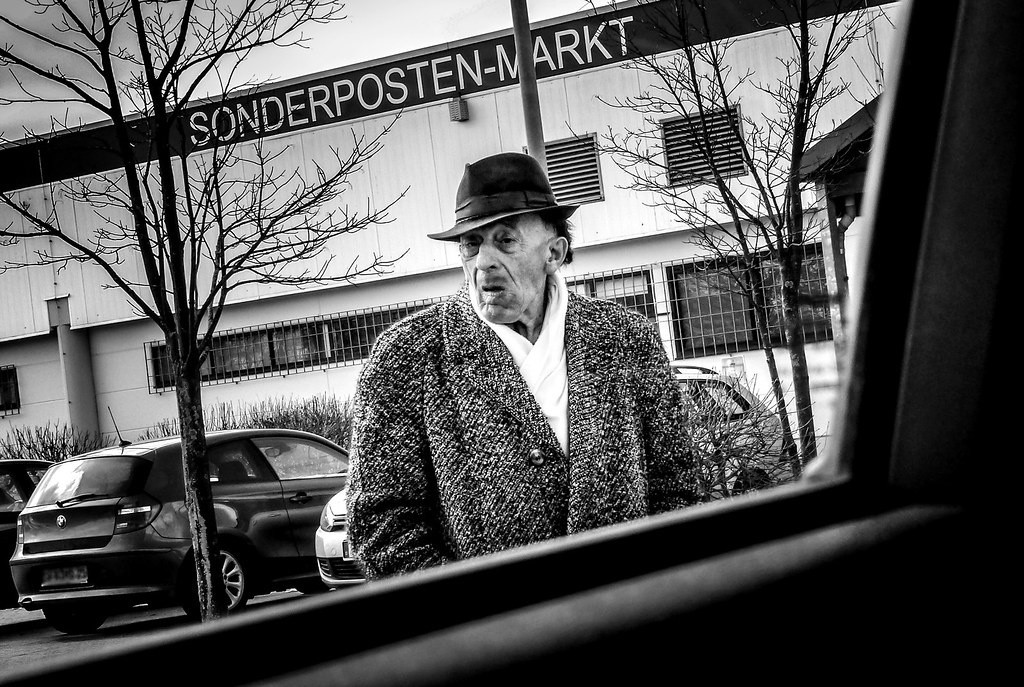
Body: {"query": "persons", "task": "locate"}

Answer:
[344,149,706,588]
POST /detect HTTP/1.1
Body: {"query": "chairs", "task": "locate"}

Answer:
[41,458,253,505]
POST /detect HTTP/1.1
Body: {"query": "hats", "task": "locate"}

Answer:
[427,153,580,242]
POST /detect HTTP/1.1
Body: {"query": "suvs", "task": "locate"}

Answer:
[666,366,789,502]
[8,428,354,637]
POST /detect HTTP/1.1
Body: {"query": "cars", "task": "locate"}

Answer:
[0,459,66,610]
